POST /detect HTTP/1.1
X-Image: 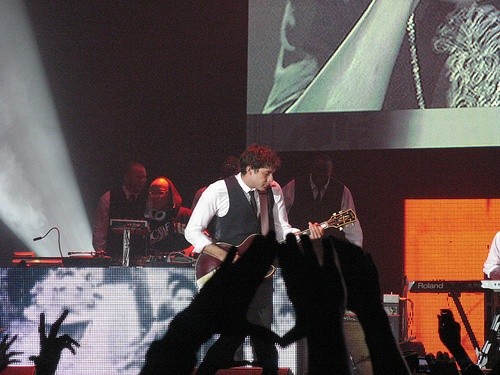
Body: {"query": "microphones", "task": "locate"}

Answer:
[271,184,277,187]
[350,356,356,369]
[33,227,63,261]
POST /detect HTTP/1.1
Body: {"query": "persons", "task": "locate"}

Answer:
[0,233,485,375]
[92,156,240,258]
[184,145,325,375]
[282,143,363,248]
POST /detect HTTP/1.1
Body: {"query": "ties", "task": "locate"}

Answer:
[130,195,135,200]
[316,190,321,201]
[249,191,257,217]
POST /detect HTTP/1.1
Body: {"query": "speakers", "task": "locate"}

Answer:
[343,304,400,375]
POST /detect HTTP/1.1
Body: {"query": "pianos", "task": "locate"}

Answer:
[408,278,500,294]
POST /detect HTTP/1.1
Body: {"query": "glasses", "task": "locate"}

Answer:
[149,191,167,198]
[223,162,239,170]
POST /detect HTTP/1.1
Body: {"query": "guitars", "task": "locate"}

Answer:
[195,207,357,295]
